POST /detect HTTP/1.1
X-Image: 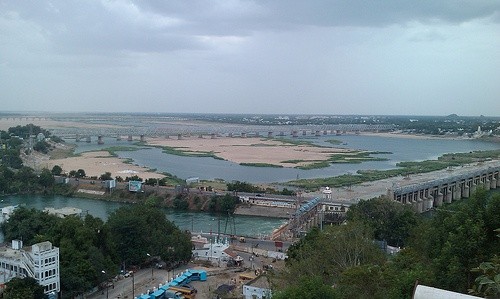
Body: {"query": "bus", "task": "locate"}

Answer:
[171,286,195,298]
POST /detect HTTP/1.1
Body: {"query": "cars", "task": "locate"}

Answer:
[256,194,264,197]
[121,270,133,278]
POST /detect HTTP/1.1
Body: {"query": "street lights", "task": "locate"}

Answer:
[147,254,153,279]
[102,270,109,298]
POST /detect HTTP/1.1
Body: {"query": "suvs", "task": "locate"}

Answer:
[179,284,197,293]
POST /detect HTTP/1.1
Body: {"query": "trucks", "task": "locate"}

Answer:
[165,290,185,299]
